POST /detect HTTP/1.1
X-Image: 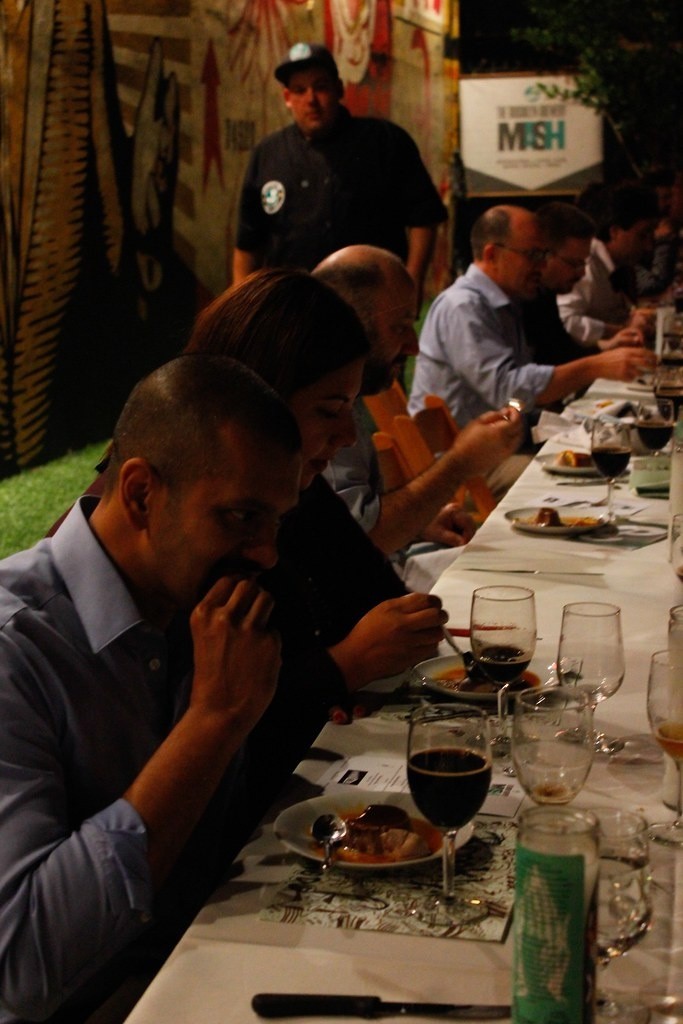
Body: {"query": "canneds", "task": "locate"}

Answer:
[508,804,603,1024]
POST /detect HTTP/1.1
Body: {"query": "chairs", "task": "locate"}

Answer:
[362,373,498,541]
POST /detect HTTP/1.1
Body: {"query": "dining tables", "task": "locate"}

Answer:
[157,369,683,1024]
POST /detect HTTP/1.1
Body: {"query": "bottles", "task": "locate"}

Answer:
[511,805,601,1024]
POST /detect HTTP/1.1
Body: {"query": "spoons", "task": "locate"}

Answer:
[313,813,347,867]
[441,625,473,671]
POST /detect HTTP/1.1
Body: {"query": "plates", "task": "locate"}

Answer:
[537,453,597,473]
[274,791,475,868]
[413,655,560,700]
[505,506,610,534]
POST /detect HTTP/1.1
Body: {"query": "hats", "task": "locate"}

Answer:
[275,42,338,88]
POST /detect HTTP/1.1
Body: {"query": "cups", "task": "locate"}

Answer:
[511,686,596,806]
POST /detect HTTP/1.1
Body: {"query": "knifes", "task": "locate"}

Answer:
[251,993,510,1020]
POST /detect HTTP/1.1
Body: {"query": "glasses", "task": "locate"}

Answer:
[495,241,551,263]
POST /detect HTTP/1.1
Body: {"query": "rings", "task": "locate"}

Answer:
[415,647,422,664]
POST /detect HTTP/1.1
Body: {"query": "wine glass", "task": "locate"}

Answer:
[647,513,683,849]
[592,422,632,519]
[407,704,492,925]
[557,602,625,753]
[654,312,683,422]
[470,585,537,755]
[635,400,674,456]
[586,806,651,1024]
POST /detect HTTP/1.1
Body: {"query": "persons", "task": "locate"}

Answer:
[182,263,450,913]
[0,348,305,1024]
[406,198,658,513]
[309,243,524,581]
[230,40,448,396]
[556,158,683,349]
